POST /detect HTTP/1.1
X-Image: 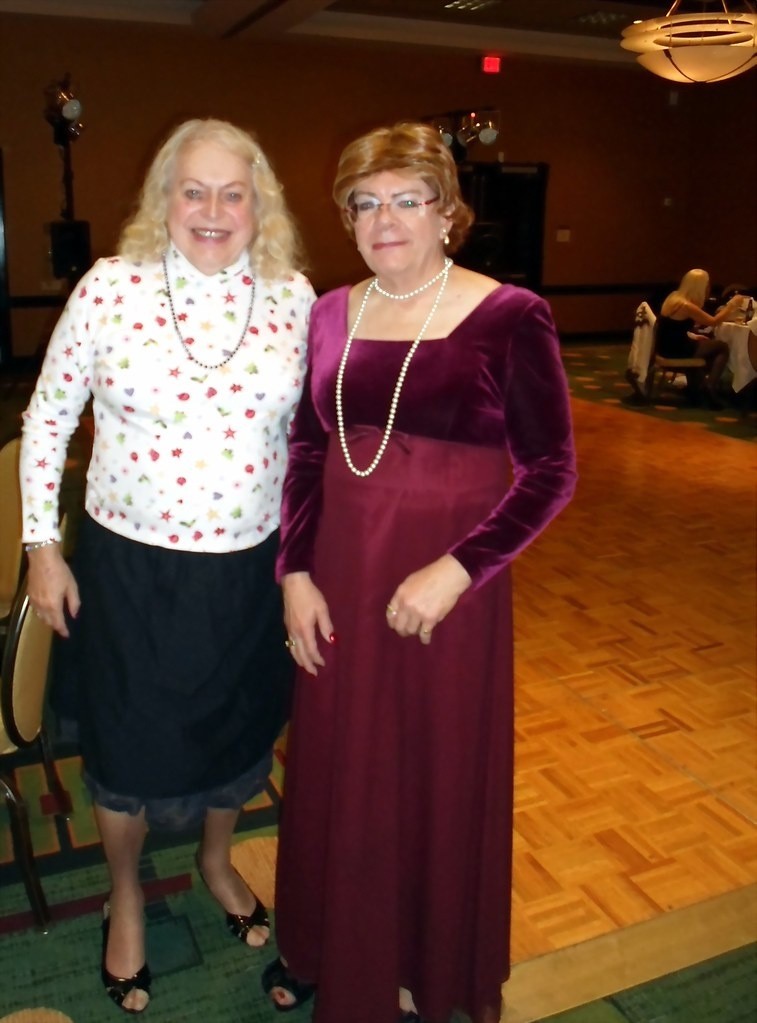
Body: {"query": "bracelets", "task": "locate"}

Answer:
[26,542,50,552]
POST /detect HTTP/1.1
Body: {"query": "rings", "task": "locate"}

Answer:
[285,640,295,647]
[387,605,397,616]
[421,628,432,635]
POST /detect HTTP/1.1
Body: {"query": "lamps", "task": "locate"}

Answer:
[621,0,757,92]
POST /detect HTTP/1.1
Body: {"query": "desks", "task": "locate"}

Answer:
[715,321,757,403]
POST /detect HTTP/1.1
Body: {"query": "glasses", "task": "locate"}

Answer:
[346,190,440,224]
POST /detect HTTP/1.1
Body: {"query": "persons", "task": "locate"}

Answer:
[660,269,745,397]
[23,118,319,1007]
[262,126,580,1023]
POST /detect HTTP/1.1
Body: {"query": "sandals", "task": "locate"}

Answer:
[194,849,272,948]
[260,955,314,1012]
[101,900,153,1013]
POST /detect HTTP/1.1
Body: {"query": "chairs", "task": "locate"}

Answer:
[0,436,69,936]
[640,302,706,404]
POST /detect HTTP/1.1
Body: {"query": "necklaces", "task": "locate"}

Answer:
[161,250,255,367]
[336,254,455,475]
